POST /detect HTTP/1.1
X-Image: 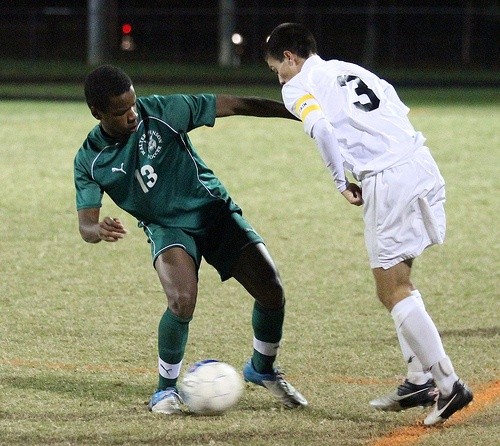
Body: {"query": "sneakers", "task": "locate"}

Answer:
[148,387,183,415]
[369,376,438,411]
[423,378,473,425]
[242,357,309,409]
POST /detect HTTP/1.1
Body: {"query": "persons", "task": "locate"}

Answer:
[72,66,310,415]
[263,21,476,427]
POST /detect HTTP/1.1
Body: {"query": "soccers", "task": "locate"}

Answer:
[180,359,246,415]
